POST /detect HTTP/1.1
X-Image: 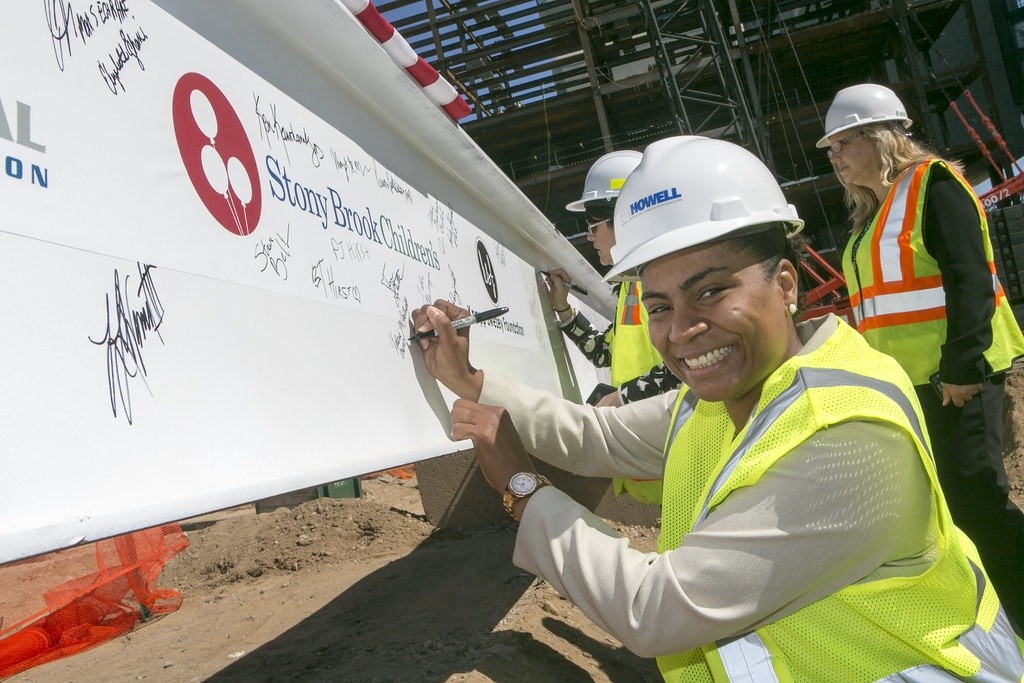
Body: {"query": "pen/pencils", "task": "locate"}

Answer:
[539,270,588,296]
[407,307,509,342]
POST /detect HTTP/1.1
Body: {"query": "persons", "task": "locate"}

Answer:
[411,136,1024,683]
[816,83,1024,640]
[544,150,683,507]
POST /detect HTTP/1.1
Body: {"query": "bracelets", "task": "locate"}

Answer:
[553,304,571,313]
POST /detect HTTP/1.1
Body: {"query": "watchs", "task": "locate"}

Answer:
[502,472,553,522]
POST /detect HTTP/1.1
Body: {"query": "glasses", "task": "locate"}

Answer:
[826,129,867,159]
[585,218,611,235]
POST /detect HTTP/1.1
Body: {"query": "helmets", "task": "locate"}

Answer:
[566,150,644,213]
[602,136,805,283]
[815,84,912,149]
